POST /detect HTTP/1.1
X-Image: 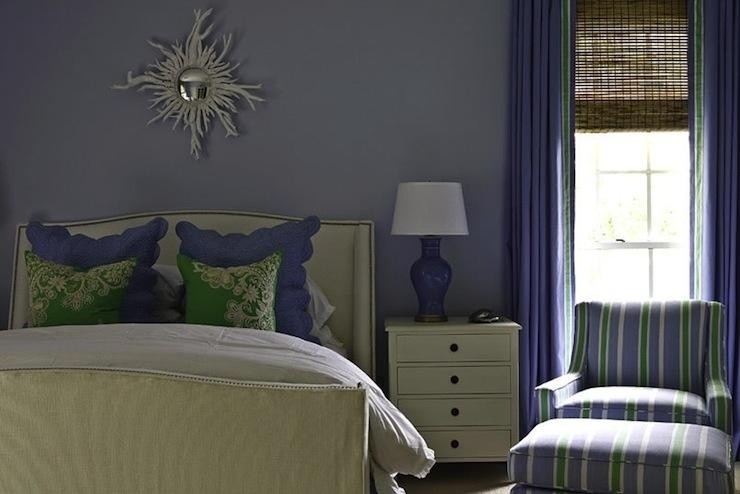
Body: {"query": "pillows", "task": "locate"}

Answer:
[175,253,281,330]
[24,220,168,323]
[176,215,320,335]
[22,248,137,328]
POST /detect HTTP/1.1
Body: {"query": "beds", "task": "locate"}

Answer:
[0,205,383,493]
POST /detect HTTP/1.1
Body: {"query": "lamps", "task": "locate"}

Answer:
[389,178,473,323]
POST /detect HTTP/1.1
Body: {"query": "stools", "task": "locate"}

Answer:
[504,416,733,494]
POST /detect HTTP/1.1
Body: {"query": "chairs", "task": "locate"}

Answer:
[507,299,738,493]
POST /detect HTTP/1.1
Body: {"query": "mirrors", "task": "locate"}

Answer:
[109,3,266,161]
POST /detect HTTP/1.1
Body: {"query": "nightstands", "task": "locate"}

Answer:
[379,318,522,478]
